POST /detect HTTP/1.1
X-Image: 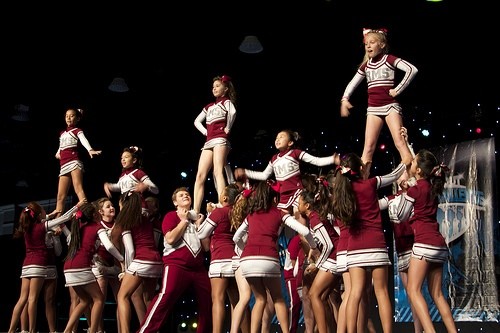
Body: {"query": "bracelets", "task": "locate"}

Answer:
[341,99,348,101]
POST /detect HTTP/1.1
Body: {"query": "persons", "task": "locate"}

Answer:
[185,75,237,220]
[341,28,418,186]
[52,108,101,232]
[9,131,457,333]
